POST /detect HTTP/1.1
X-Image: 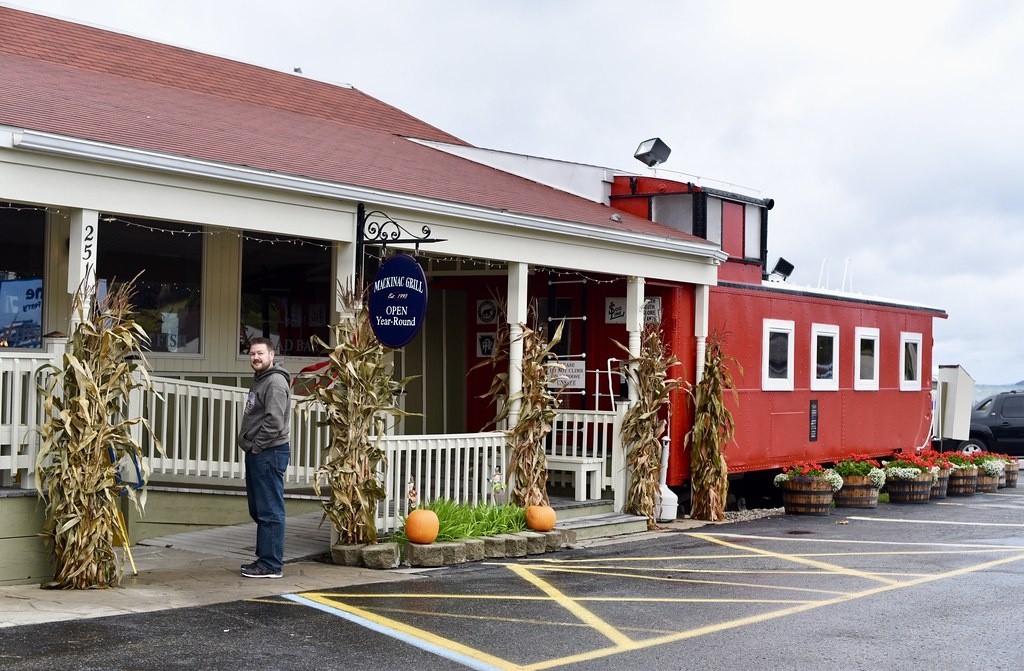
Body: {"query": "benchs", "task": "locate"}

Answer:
[486,458,604,501]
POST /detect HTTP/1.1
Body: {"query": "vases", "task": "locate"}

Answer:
[781,480,833,516]
[976,468,998,492]
[1006,464,1019,487]
[930,468,950,499]
[999,468,1006,488]
[886,473,931,504]
[949,468,978,496]
[833,476,880,508]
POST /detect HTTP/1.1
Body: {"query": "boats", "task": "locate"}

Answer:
[0,319,41,348]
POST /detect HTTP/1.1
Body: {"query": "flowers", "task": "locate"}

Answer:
[775,449,1019,493]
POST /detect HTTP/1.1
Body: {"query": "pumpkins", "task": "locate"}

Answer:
[406,505,439,544]
[525,502,556,532]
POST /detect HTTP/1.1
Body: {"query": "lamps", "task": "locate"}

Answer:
[762,256,794,282]
[634,137,671,177]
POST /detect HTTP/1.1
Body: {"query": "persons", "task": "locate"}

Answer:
[236,337,291,578]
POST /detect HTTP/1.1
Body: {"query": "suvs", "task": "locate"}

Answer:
[932,389,1024,458]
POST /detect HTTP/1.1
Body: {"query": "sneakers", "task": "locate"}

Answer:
[241,559,260,569]
[241,563,283,578]
